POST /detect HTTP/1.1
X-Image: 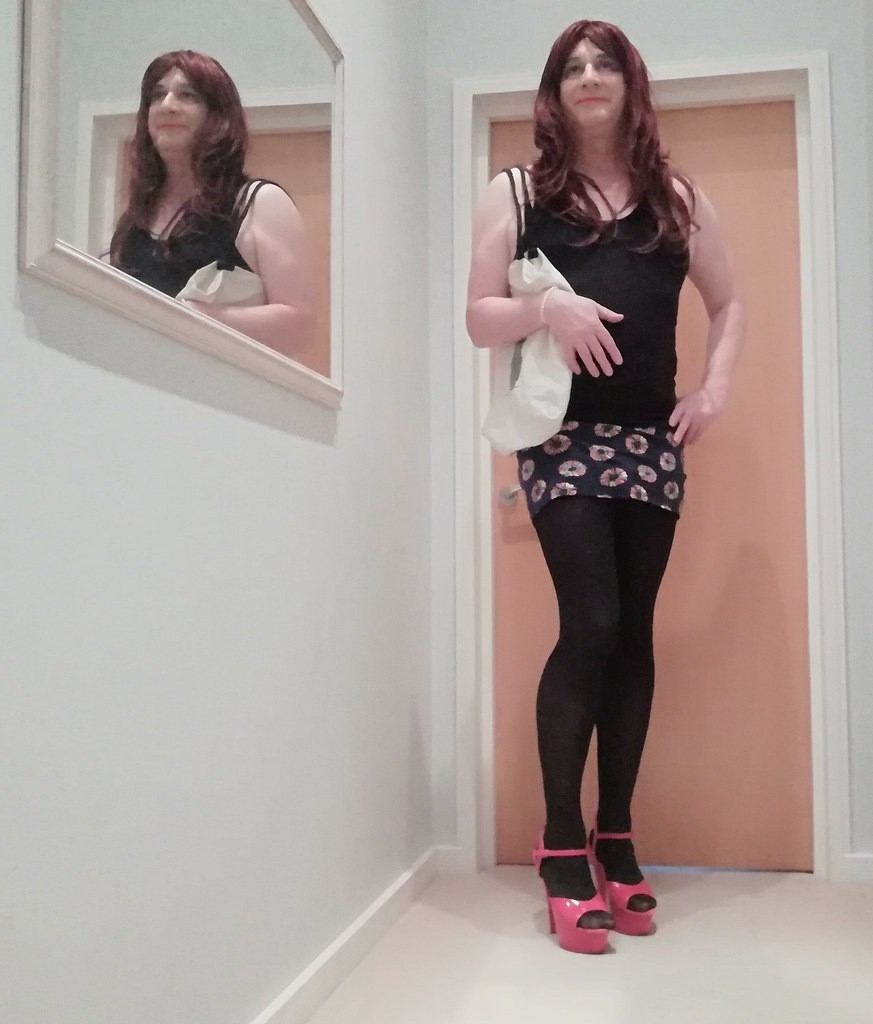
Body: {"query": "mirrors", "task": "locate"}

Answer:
[16,0,347,410]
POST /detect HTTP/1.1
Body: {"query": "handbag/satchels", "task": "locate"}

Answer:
[488,166,577,455]
[174,174,271,306]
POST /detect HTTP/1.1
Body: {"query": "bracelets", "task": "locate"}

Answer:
[540,285,558,323]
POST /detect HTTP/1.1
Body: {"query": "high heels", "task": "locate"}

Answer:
[585,826,656,935]
[531,829,609,954]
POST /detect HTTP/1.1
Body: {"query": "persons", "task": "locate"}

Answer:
[465,20,747,955]
[98,49,317,368]
